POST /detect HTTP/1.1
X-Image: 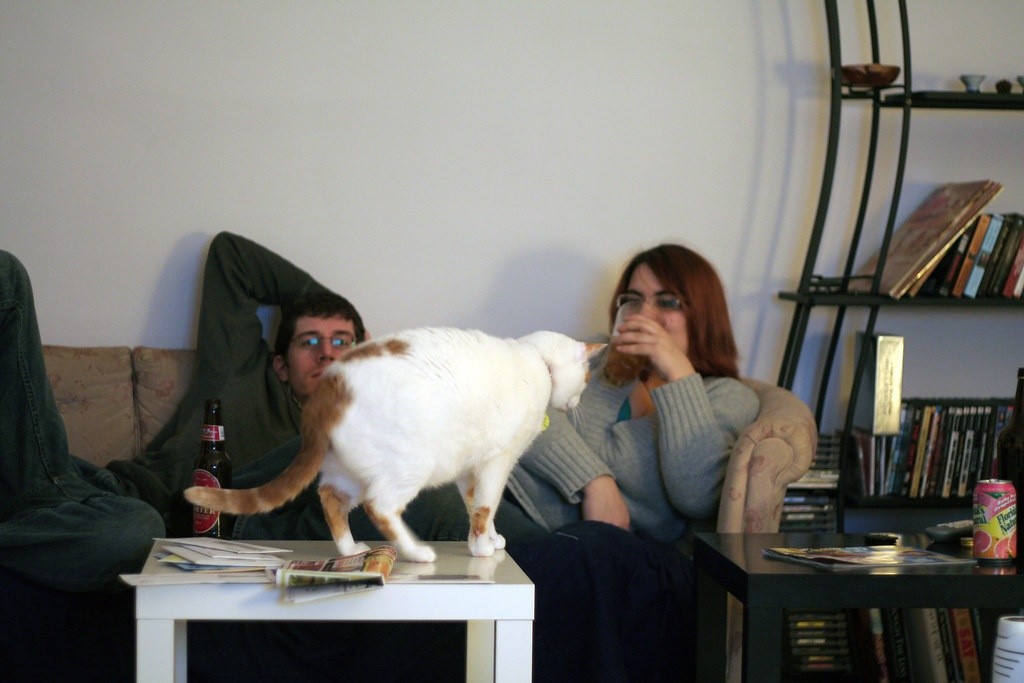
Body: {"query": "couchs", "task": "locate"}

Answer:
[41,346,819,683]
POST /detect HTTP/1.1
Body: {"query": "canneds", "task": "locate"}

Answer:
[973,478,1018,562]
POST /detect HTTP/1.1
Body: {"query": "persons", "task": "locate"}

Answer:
[0,230,366,594]
[210,243,759,548]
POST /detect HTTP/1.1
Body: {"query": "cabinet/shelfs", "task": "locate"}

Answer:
[731,1,1023,682]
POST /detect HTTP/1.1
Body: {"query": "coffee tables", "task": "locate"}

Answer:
[124,539,536,683]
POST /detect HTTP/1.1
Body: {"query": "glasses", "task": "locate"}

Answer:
[288,334,357,350]
[617,293,684,313]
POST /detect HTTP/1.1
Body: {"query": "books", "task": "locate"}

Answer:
[781,603,994,683]
[779,434,842,532]
[849,331,904,435]
[848,179,1024,299]
[838,397,1018,499]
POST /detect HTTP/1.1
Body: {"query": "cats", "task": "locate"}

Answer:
[183,325,611,566]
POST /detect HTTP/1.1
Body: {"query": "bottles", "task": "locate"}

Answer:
[192,398,231,543]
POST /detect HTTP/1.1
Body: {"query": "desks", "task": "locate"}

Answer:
[690,533,1024,683]
